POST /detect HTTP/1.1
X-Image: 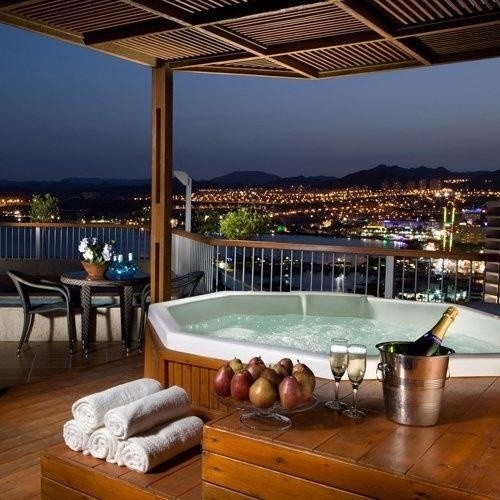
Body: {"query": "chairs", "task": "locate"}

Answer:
[7,269,77,360]
[136,269,205,352]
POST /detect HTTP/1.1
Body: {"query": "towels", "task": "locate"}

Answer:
[63,374,205,467]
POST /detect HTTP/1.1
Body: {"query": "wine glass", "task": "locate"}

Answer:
[215,390,320,433]
[342,343,368,418]
[325,338,349,410]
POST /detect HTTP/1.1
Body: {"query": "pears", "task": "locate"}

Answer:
[214,356,316,408]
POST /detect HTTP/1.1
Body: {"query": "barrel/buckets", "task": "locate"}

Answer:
[375,341,455,426]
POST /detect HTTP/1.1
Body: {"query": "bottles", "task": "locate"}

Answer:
[105,253,139,281]
[404,306,458,357]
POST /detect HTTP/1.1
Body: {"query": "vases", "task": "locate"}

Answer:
[79,258,106,280]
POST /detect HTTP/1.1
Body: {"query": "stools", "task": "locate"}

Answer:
[39,437,204,500]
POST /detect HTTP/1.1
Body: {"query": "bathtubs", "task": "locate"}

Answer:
[148,290,500,359]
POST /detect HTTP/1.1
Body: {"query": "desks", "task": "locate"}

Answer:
[60,269,151,359]
[203,378,500,499]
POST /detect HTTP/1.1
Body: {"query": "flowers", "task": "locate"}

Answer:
[77,234,121,266]
